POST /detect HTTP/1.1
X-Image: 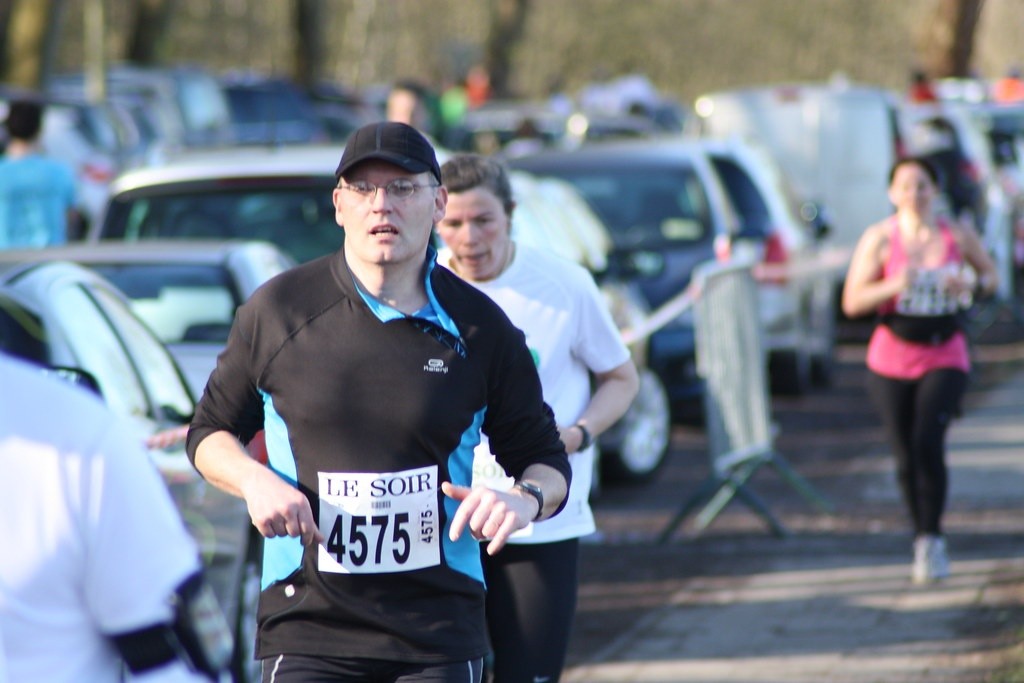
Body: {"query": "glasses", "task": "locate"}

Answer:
[338,179,440,200]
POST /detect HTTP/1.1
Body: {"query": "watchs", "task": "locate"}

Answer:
[513,482,544,521]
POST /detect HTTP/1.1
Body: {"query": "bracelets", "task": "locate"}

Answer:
[974,277,983,298]
[575,424,589,452]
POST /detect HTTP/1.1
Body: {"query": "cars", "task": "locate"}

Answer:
[0,62,1023,682]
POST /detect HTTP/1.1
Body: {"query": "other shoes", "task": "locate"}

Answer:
[911,535,949,580]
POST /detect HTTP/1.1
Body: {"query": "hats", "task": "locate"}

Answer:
[335,121,442,186]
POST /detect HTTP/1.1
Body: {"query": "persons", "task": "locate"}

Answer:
[997,66,1024,104]
[1,101,104,252]
[842,158,998,584]
[908,72,936,103]
[436,155,640,682]
[0,347,206,683]
[440,65,490,150]
[186,123,572,683]
[387,80,422,124]
